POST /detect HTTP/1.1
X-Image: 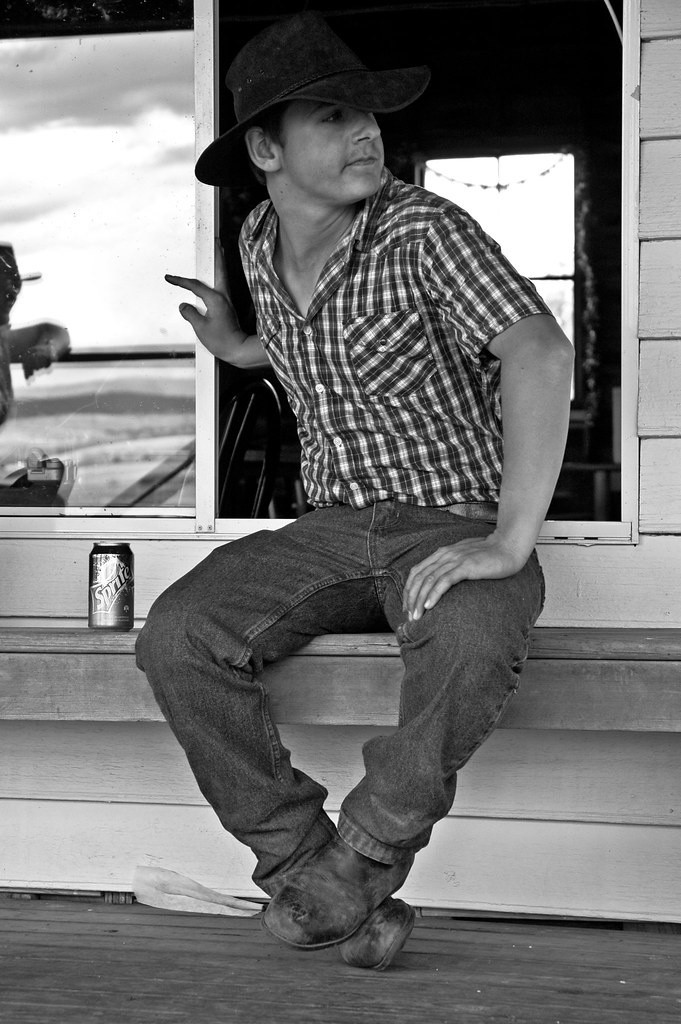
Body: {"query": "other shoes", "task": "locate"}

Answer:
[263,833,410,951]
[335,896,414,970]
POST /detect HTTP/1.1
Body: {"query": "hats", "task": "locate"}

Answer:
[195,15,431,188]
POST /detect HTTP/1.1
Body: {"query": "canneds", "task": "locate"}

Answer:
[88,541,134,632]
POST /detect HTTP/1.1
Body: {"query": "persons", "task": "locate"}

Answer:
[132,15,576,969]
[0,243,72,426]
